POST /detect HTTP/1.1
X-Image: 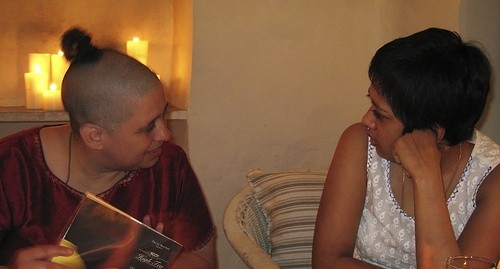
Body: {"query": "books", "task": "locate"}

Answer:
[50,192,183,269]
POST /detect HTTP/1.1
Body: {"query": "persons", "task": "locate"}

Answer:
[0,27,219,269]
[311,27,500,269]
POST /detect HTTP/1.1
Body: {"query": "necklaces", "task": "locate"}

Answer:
[65,132,72,184]
[401,143,462,216]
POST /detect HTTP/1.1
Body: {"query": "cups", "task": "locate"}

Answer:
[445,256,496,269]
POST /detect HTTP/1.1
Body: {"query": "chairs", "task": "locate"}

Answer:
[222,166,328,269]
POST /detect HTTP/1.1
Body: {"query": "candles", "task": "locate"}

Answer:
[24,36,160,110]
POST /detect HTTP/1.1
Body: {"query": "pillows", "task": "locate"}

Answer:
[244,167,329,269]
